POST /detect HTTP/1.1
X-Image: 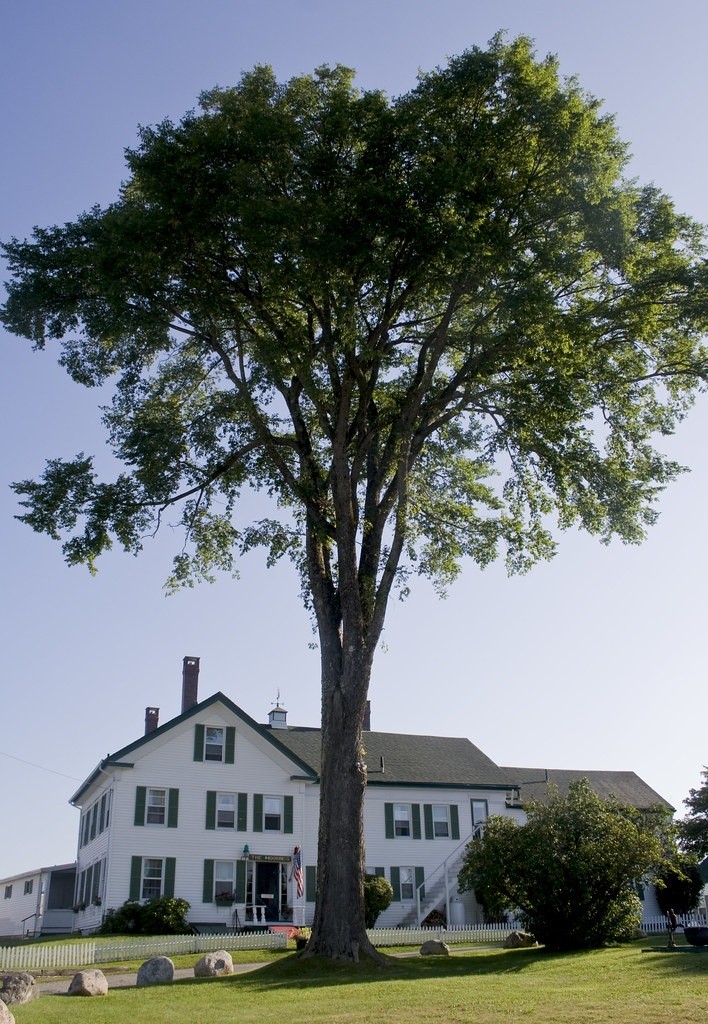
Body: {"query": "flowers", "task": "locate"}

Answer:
[289,926,312,942]
[78,901,86,907]
[214,890,236,901]
[72,905,79,910]
[92,895,101,901]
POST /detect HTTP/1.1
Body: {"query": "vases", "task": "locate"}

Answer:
[215,900,233,907]
[296,939,308,950]
[683,927,708,946]
[93,900,101,906]
[79,906,85,910]
[73,910,79,913]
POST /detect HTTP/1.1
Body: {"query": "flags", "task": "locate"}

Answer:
[293,847,304,899]
